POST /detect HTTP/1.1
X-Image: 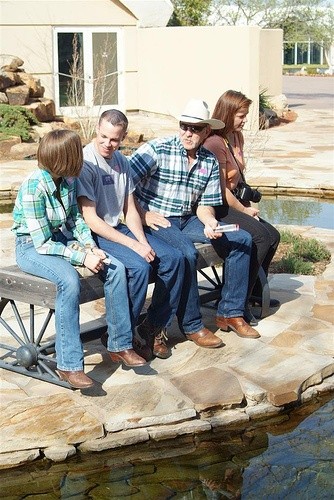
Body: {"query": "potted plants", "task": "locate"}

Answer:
[259,88,272,127]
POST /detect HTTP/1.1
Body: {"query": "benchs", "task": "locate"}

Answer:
[0,243,224,391]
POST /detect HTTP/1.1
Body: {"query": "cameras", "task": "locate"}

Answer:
[231,182,261,203]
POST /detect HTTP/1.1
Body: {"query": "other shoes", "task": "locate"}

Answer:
[249,295,280,306]
[243,309,258,326]
[101,333,108,347]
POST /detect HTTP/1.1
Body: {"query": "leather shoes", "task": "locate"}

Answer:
[185,326,223,348]
[215,316,260,338]
[57,368,94,388]
[153,328,172,358]
[108,349,147,367]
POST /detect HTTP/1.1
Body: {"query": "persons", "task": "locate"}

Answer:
[128,99,261,348]
[10,130,148,389]
[77,109,183,359]
[205,475,243,500]
[205,89,281,325]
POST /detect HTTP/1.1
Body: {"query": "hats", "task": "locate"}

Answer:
[180,101,225,129]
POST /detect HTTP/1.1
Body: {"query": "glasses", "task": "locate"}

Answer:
[180,123,206,133]
